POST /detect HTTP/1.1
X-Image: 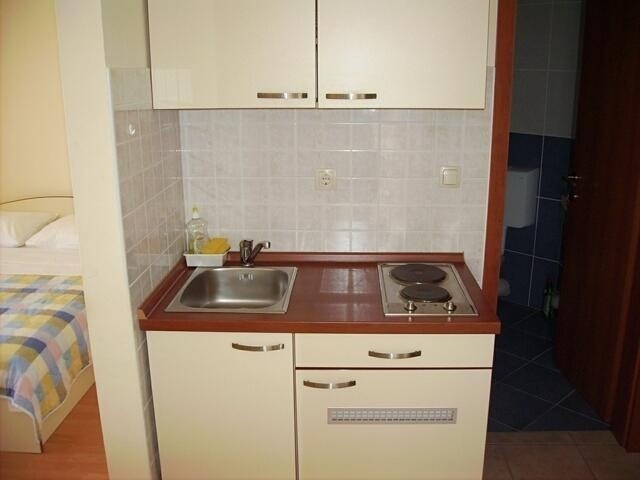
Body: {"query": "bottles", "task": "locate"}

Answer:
[541,278,557,320]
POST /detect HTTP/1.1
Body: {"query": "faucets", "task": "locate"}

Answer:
[239,238,271,266]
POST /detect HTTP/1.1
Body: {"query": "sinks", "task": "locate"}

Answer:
[166,265,297,313]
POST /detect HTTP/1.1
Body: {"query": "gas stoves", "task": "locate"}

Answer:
[376,260,481,318]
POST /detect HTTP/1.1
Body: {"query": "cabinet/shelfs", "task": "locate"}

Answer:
[144,330,496,480]
[146,1,490,110]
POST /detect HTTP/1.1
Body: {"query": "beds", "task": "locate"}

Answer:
[0,196,95,454]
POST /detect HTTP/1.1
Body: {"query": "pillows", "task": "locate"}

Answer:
[0,212,78,248]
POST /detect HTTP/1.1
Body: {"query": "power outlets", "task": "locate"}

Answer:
[314,169,337,191]
